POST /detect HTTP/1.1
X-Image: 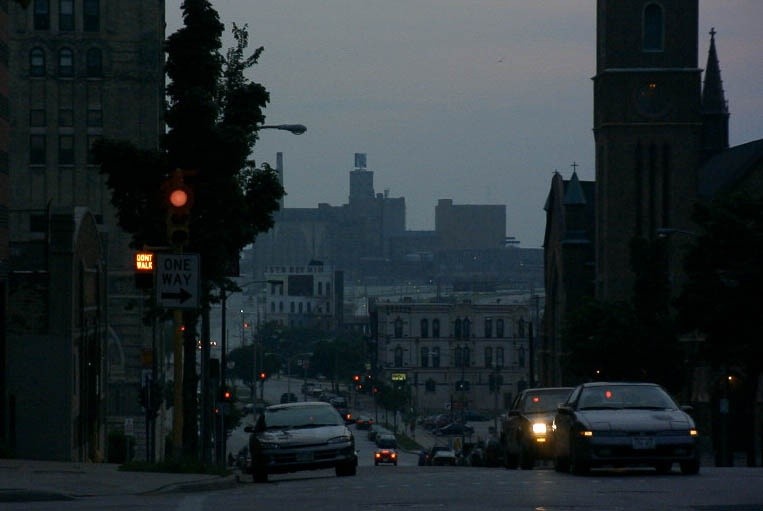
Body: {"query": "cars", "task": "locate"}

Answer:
[416,414,475,437]
[244,402,358,483]
[418,443,498,467]
[355,416,396,448]
[375,448,397,466]
[553,382,701,477]
[504,387,575,471]
[300,381,353,425]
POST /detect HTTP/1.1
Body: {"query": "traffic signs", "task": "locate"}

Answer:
[155,253,199,309]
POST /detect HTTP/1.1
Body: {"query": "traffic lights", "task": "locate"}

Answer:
[165,185,193,248]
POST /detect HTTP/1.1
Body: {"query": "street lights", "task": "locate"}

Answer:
[221,279,284,469]
[182,122,309,469]
